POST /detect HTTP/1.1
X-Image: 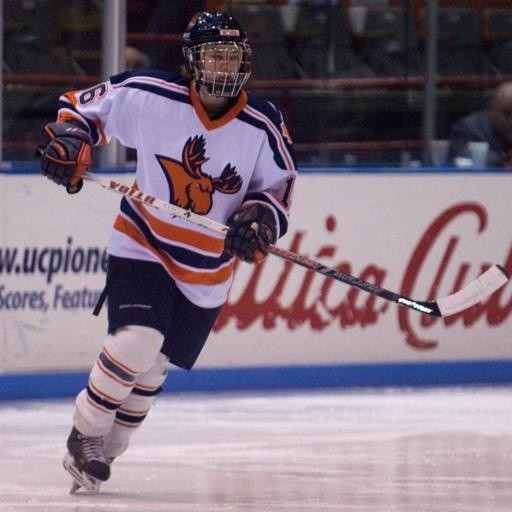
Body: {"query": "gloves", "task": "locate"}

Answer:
[223,203,280,266]
[39,122,93,193]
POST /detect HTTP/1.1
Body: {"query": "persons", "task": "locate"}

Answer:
[37,7,298,480]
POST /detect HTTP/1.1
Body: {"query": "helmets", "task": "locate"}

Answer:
[181,12,255,97]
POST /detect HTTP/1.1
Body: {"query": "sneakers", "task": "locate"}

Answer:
[66,426,110,482]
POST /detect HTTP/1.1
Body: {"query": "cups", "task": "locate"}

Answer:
[430,139,451,167]
[467,140,491,168]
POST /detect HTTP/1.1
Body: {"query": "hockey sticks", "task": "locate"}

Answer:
[35,146,509,317]
